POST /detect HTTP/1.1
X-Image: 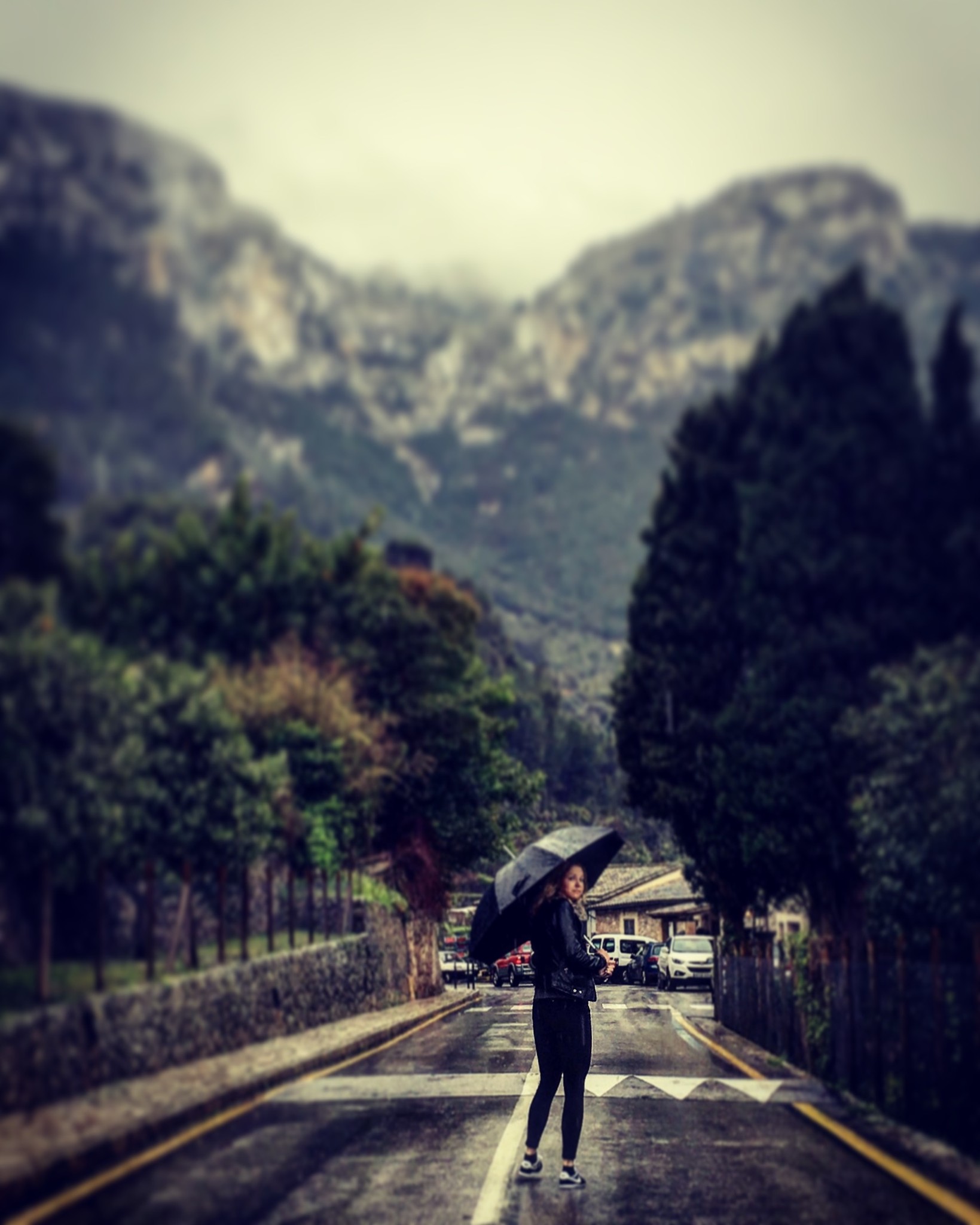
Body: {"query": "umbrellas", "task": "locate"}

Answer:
[466,825,627,980]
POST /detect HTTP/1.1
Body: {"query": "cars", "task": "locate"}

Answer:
[625,941,667,986]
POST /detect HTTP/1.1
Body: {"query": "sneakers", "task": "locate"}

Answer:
[558,1171,586,1190]
[518,1155,543,1177]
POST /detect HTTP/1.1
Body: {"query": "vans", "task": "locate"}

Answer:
[587,933,660,984]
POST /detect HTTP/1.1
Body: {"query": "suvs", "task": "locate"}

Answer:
[657,934,718,992]
[438,950,479,984]
[488,940,538,988]
[439,941,491,978]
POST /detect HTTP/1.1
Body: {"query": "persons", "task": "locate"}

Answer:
[518,858,616,1190]
